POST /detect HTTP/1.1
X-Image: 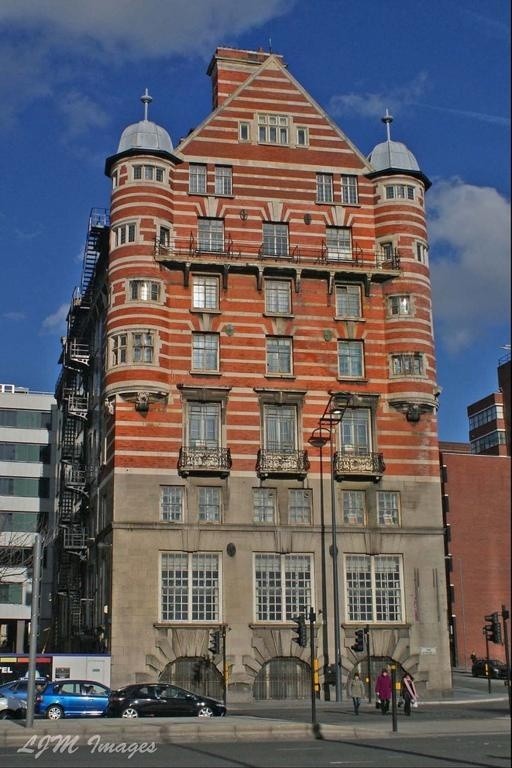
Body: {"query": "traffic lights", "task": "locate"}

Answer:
[351,631,364,651]
[291,613,307,646]
[484,612,501,644]
[208,631,220,654]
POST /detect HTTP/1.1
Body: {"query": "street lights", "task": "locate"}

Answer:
[318,392,352,702]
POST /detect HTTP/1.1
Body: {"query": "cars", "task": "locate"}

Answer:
[1,678,227,717]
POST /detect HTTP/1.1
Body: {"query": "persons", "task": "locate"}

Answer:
[349,672,365,716]
[400,673,414,716]
[470,650,476,662]
[375,668,392,715]
[87,685,101,694]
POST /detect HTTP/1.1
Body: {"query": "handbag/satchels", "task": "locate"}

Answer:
[375,701,381,709]
[397,701,405,708]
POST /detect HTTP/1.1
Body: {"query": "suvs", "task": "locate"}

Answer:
[472,658,507,677]
